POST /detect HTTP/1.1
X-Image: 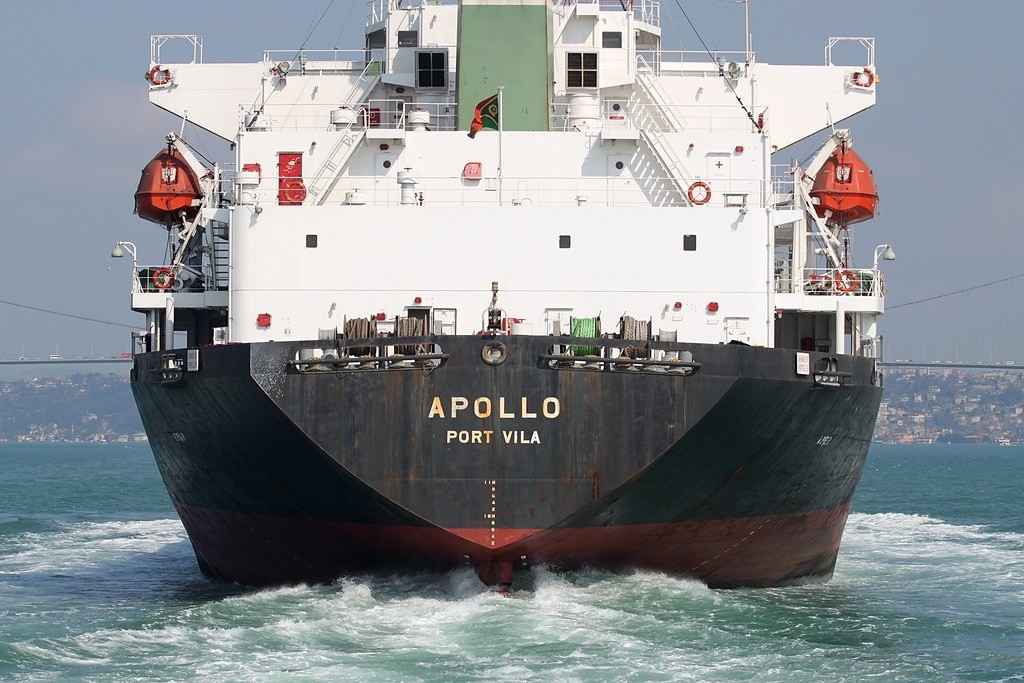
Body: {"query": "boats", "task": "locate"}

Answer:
[111,0,897,595]
[134,148,203,226]
[809,146,879,229]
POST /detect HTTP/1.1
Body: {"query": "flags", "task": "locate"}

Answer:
[467,93,499,139]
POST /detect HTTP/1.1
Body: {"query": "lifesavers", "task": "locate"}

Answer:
[836,271,857,291]
[854,68,874,87]
[153,268,175,288]
[687,182,711,206]
[150,64,171,85]
[171,278,184,291]
